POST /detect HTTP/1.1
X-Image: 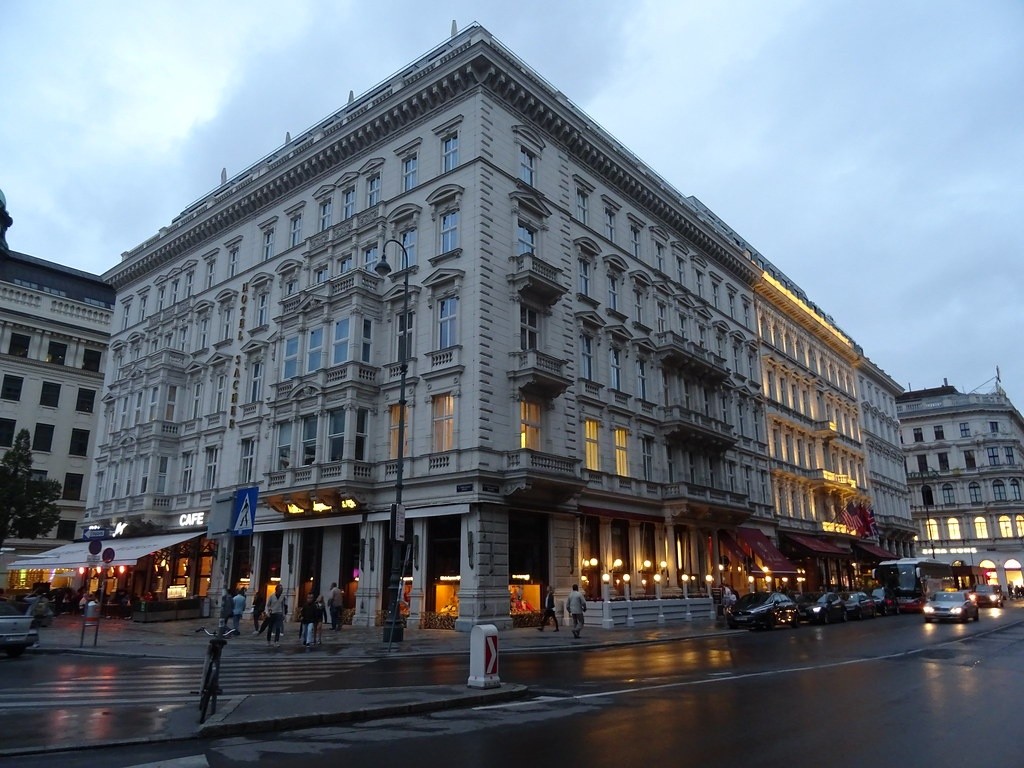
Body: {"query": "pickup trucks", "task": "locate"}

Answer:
[0,603,39,658]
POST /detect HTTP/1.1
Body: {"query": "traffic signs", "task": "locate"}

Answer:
[83,529,110,540]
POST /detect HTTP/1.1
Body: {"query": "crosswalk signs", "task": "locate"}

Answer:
[228,486,260,537]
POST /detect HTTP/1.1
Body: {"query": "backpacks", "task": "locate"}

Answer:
[31,600,49,616]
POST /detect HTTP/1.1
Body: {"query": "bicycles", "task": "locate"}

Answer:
[191,626,241,724]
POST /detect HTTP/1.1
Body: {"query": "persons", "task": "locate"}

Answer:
[266,584,287,648]
[301,594,317,648]
[314,595,327,645]
[0,584,154,617]
[565,584,587,638]
[252,592,265,635]
[700,582,820,603]
[327,582,343,631]
[1008,584,1024,601]
[257,617,269,635]
[218,586,247,636]
[536,585,559,632]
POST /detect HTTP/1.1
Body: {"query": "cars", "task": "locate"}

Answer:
[795,592,848,625]
[923,588,979,624]
[843,592,877,620]
[867,589,887,615]
[726,592,798,632]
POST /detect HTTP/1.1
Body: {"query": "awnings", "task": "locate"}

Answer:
[716,529,901,578]
[6,530,207,571]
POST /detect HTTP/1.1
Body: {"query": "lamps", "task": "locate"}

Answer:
[748,566,807,582]
[581,558,712,585]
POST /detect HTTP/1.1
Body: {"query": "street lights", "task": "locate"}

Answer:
[376,238,413,641]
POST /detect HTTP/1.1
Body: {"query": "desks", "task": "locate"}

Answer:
[106,603,119,618]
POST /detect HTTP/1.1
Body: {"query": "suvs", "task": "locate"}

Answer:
[975,584,1003,608]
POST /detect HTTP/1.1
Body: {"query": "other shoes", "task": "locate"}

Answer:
[279,632,285,636]
[553,629,559,632]
[538,628,544,631]
[572,630,582,639]
[274,642,280,647]
[305,643,311,647]
[252,631,259,634]
[271,632,276,636]
[302,640,306,647]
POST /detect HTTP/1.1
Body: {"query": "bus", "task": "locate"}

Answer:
[878,557,955,614]
